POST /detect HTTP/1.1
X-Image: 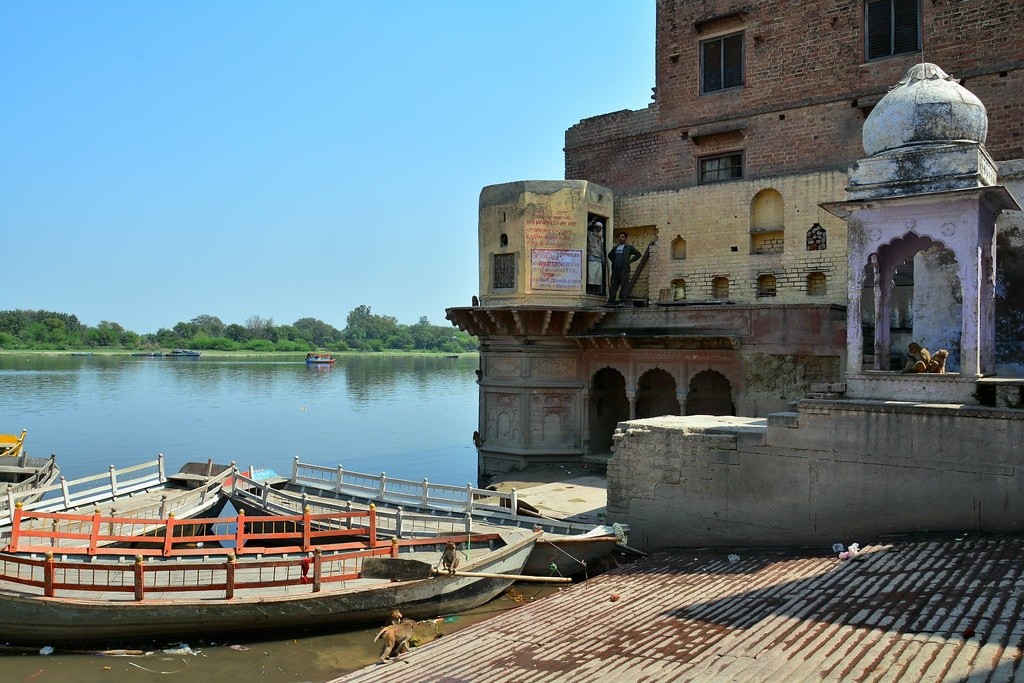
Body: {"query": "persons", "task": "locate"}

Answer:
[586,216,604,292]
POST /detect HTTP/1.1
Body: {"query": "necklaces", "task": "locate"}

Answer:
[607,232,641,308]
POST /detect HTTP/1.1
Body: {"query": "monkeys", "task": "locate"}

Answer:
[475,369,482,379]
[597,555,622,572]
[901,342,949,374]
[373,610,418,664]
[434,540,461,573]
[473,431,485,448]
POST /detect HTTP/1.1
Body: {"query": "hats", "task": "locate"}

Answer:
[592,222,603,236]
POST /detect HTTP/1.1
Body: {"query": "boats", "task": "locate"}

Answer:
[0,428,27,459]
[3,499,545,647]
[217,455,625,579]
[0,454,240,553]
[164,348,203,357]
[0,451,62,520]
[305,353,336,364]
[131,350,164,357]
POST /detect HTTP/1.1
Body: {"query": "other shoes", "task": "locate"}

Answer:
[618,300,625,306]
[607,300,616,305]
